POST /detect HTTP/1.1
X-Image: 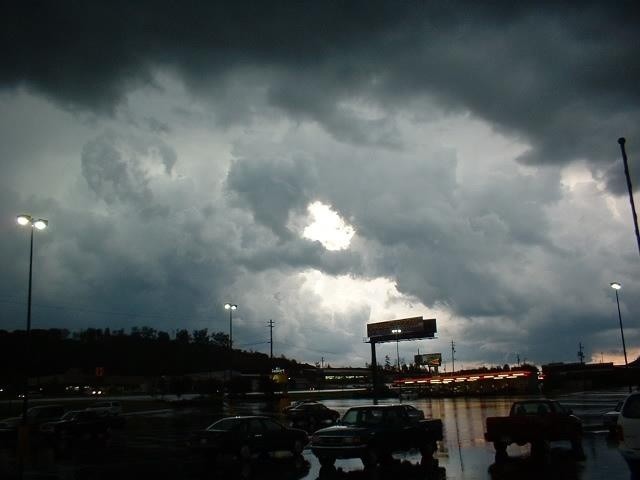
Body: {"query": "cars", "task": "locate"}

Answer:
[602,390,640,461]
[190,400,442,465]
[18,390,46,400]
[401,391,417,400]
[1,401,123,437]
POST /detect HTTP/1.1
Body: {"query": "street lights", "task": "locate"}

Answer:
[610,282,628,368]
[392,324,402,367]
[17,214,48,480]
[224,303,237,347]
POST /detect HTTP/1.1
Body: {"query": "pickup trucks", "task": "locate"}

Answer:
[484,399,584,450]
